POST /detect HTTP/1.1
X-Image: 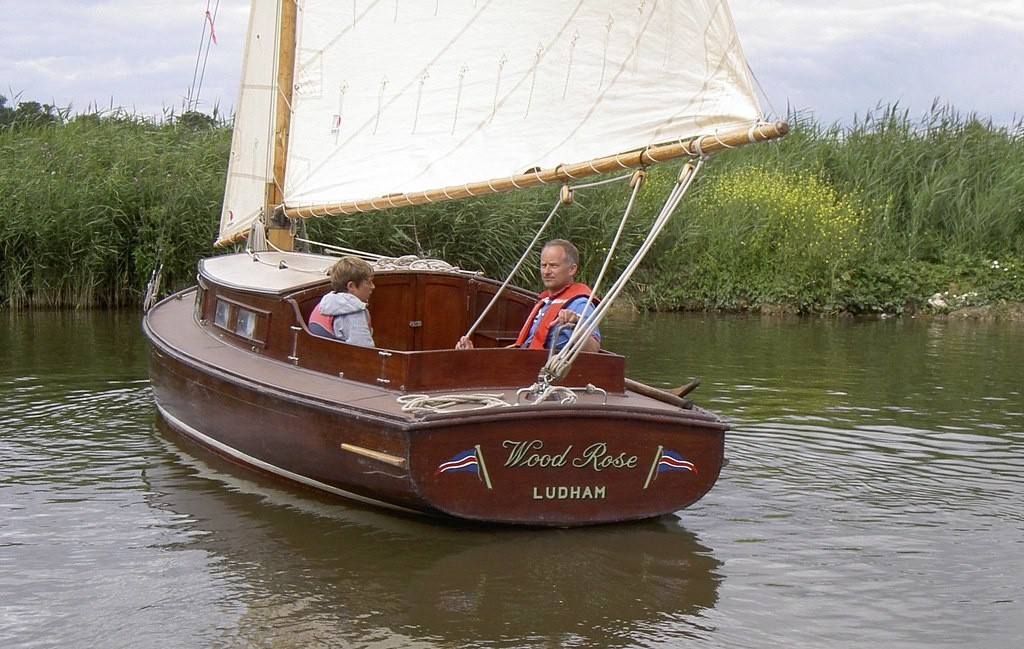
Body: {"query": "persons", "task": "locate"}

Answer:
[308,256,378,349]
[454,239,601,353]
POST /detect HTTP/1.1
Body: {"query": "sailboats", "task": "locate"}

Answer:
[141,0,793,529]
